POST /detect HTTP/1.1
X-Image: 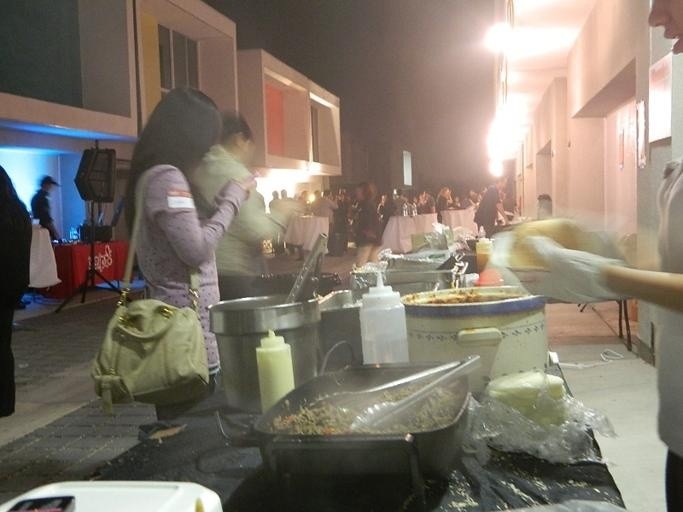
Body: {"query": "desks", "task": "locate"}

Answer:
[37,239,131,298]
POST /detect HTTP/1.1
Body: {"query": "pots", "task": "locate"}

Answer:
[400,282,548,406]
[258,362,472,491]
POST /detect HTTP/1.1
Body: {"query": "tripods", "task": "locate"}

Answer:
[52,201,133,313]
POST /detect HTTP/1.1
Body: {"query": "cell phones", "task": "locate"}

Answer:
[238,171,260,186]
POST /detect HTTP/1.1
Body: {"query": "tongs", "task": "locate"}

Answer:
[304,355,479,428]
[287,233,329,304]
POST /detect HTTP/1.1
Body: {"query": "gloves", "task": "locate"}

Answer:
[511,237,630,303]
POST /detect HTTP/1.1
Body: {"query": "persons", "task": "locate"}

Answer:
[509,0,683,512]
[31,175,64,246]
[0,167,31,417]
[191,115,303,301]
[125,88,257,421]
[270,175,508,265]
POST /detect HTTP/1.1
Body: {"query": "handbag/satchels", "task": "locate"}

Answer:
[92,299,209,405]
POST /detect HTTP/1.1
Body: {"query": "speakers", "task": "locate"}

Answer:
[74,148,117,203]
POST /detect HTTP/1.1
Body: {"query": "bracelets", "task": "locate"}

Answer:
[58,238,63,244]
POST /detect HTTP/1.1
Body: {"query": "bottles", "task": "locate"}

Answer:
[402,201,417,216]
[476,230,493,269]
[257,330,295,419]
[71,228,79,245]
[358,268,409,363]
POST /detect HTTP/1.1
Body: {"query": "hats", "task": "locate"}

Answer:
[42,176,59,184]
